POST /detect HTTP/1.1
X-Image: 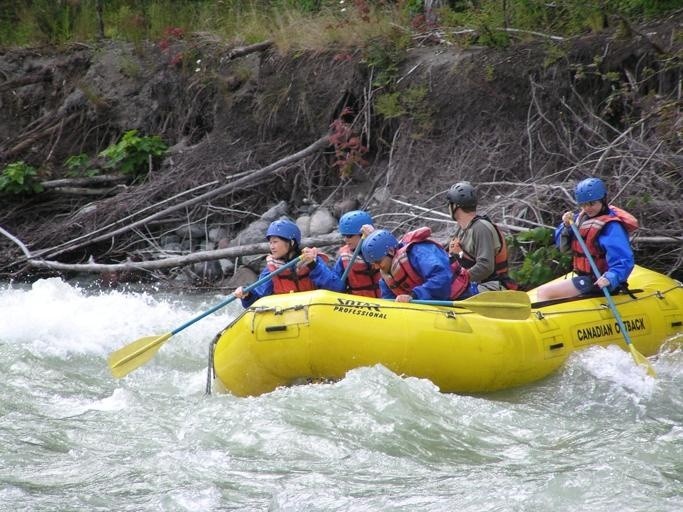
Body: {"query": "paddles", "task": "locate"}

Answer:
[397,291,529,319]
[568,218,657,379]
[109,256,302,377]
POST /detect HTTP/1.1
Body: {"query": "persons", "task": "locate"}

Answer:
[536,177,638,302]
[233,180,508,308]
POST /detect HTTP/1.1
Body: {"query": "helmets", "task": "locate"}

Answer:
[266,220,301,245]
[447,183,477,211]
[362,230,398,262]
[339,211,373,234]
[575,178,606,204]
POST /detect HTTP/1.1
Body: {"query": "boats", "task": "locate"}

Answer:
[212,258,682,396]
